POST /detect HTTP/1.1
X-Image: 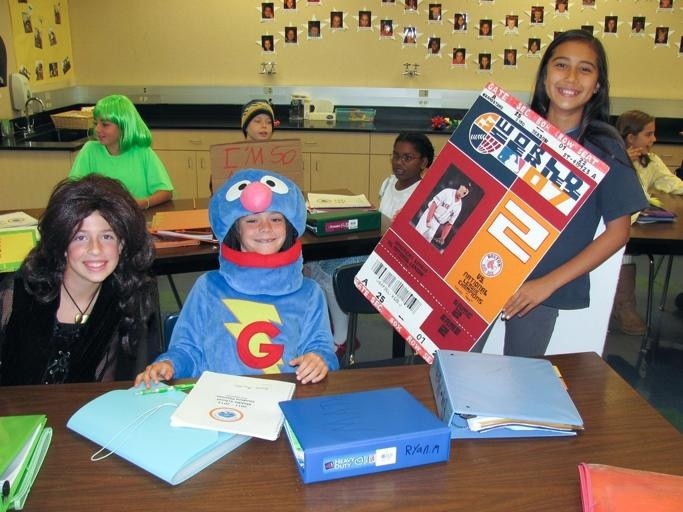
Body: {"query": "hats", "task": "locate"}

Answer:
[240,99,275,137]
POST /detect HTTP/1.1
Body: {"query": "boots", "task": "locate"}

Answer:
[612,262,648,336]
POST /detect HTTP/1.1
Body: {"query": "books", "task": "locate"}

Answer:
[308,193,371,209]
[171,371,296,441]
[637,198,678,225]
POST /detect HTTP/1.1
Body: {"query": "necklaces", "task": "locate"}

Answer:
[63,280,102,326]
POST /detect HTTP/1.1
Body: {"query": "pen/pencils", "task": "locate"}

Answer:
[153,230,220,244]
[135,383,197,397]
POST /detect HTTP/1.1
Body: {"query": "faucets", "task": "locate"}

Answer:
[269,62,278,75]
[24,97,46,134]
[257,61,268,75]
[400,62,411,77]
[410,63,421,77]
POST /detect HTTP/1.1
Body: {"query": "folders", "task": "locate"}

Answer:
[274,386,451,483]
[63,380,252,486]
[430,348,586,440]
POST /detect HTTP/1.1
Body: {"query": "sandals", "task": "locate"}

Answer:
[332,335,361,363]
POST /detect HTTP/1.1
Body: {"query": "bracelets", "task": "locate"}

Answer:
[146,198,150,207]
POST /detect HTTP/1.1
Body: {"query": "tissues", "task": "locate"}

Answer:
[308,120,337,129]
[308,98,337,121]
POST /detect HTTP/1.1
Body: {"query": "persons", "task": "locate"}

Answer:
[133,168,337,389]
[616,110,683,334]
[470,30,649,358]
[67,95,172,212]
[258,1,683,72]
[315,131,434,360]
[210,99,274,197]
[415,177,471,249]
[2,174,153,389]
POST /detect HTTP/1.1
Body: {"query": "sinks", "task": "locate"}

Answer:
[24,127,96,143]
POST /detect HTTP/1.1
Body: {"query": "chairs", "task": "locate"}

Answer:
[332,262,412,366]
[163,313,177,351]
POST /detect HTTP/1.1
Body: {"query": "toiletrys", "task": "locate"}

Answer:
[0,118,16,138]
[288,94,310,121]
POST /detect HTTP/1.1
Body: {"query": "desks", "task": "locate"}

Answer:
[623,190,682,378]
[0,188,406,359]
[0,352,683,512]
